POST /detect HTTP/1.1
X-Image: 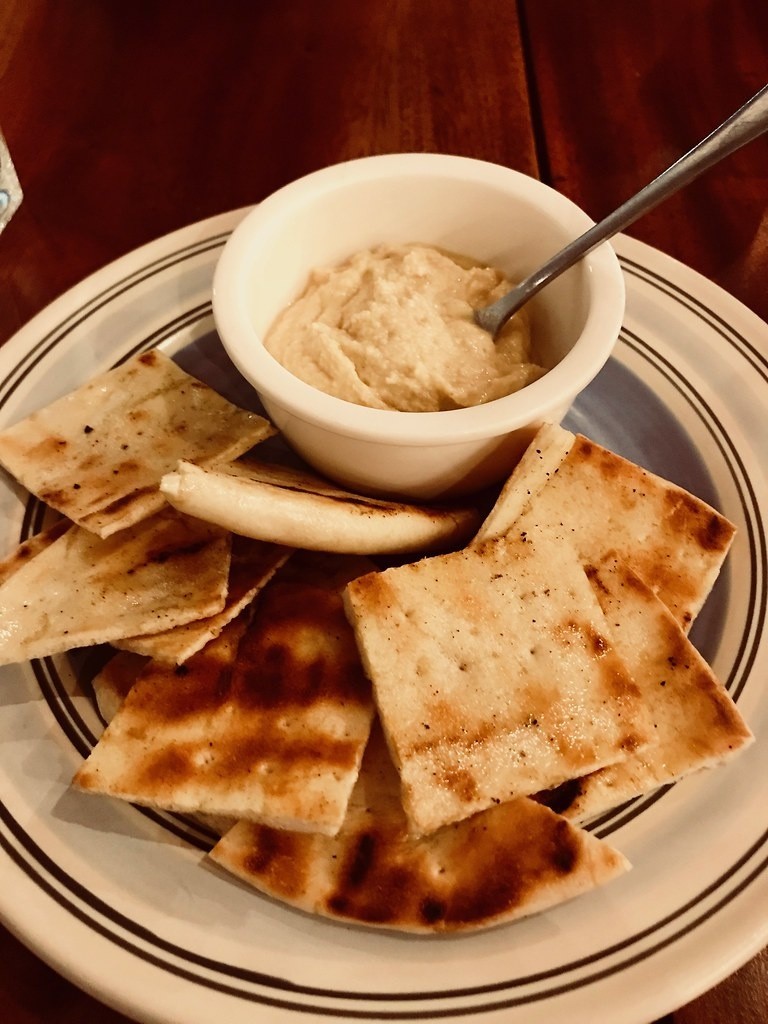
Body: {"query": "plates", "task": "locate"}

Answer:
[0,204,767,1024]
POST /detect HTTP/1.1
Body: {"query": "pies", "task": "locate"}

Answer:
[0,349,755,935]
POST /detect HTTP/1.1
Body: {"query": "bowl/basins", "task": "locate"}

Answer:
[213,153,625,503]
